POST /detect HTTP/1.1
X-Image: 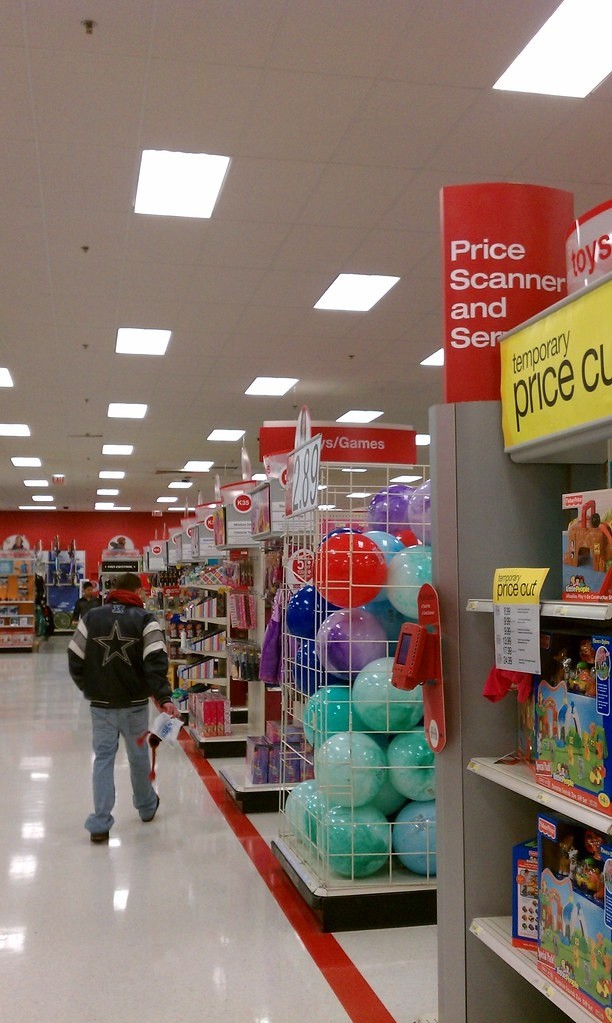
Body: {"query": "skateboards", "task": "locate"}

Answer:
[417,581,448,754]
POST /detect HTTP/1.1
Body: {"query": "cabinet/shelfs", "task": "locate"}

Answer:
[0,557,34,654]
[426,270,612,1023]
[167,559,208,720]
[178,554,230,726]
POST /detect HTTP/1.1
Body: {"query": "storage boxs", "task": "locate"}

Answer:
[192,565,223,585]
[201,693,219,737]
[184,596,218,618]
[536,811,612,1023]
[283,744,300,783]
[515,691,536,774]
[561,488,612,603]
[266,720,308,743]
[195,694,211,737]
[187,629,227,652]
[188,693,196,729]
[300,743,315,783]
[246,735,269,784]
[215,692,232,736]
[178,679,191,688]
[532,622,612,817]
[205,693,224,736]
[167,667,175,691]
[177,657,215,679]
[511,836,538,952]
[262,736,283,783]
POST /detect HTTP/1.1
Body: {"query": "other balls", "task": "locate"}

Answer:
[314,610,388,679]
[372,485,414,533]
[288,584,334,638]
[285,475,435,878]
[353,658,429,731]
[296,644,328,695]
[312,531,390,608]
[386,725,435,800]
[308,732,391,807]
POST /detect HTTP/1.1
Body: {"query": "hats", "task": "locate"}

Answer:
[136,716,186,781]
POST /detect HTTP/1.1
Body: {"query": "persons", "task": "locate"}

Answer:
[66,572,180,843]
[13,536,24,550]
[71,582,99,627]
[117,537,126,549]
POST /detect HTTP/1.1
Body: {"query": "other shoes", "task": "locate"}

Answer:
[142,794,159,822]
[90,832,109,841]
